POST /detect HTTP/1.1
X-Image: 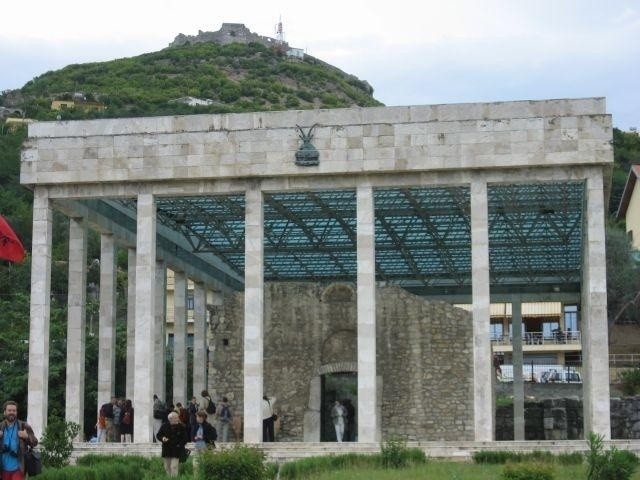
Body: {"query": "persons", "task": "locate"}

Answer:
[0,401,38,480]
[98,390,234,443]
[526,328,571,346]
[331,401,348,443]
[190,411,218,477]
[262,396,276,442]
[156,412,188,476]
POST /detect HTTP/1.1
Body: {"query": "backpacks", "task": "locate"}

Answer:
[205,396,216,414]
[179,408,187,422]
[218,404,230,422]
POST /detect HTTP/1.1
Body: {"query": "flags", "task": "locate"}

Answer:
[0,217,24,262]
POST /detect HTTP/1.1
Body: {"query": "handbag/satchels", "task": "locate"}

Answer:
[273,414,277,421]
[25,447,42,476]
[179,448,190,463]
